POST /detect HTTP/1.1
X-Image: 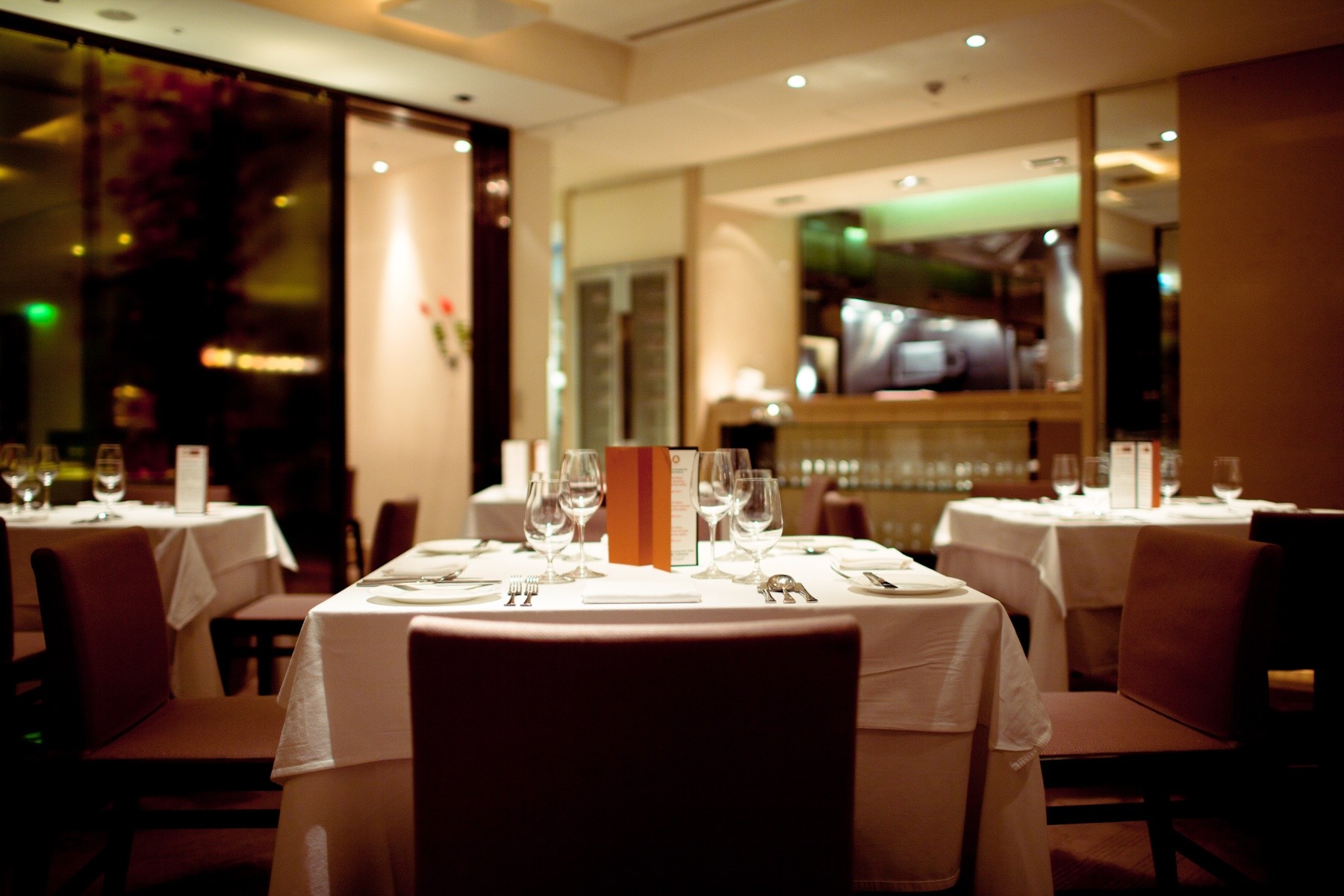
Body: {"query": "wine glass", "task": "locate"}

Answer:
[1159,453,1179,506]
[690,446,784,585]
[1211,457,1243,508]
[1052,454,1111,515]
[0,442,58,514]
[523,449,604,584]
[93,444,125,523]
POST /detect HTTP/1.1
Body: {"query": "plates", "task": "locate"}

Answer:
[372,582,502,603]
[382,562,466,577]
[418,539,482,553]
[845,569,967,594]
[777,535,852,554]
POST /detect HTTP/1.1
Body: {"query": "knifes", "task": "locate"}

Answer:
[757,582,776,603]
[863,572,898,588]
[794,583,819,602]
[357,580,502,587]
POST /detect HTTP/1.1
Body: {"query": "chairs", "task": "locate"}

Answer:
[0,422,1342,896]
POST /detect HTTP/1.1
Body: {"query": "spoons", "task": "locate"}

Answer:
[71,515,122,524]
[362,569,463,582]
[768,574,796,603]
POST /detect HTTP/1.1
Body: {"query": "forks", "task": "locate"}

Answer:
[505,573,538,608]
